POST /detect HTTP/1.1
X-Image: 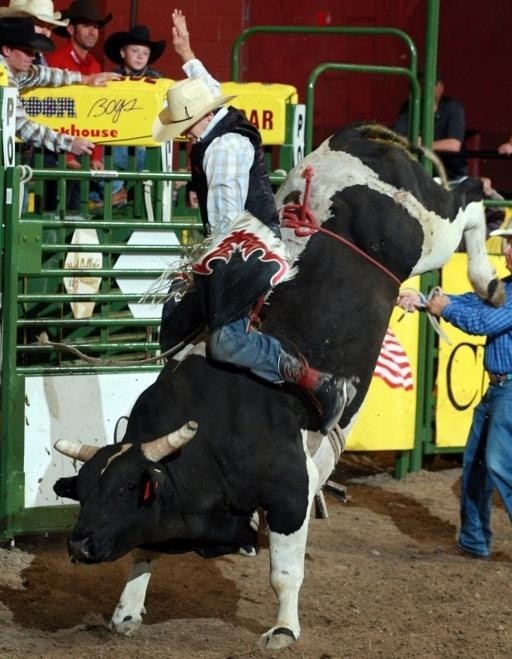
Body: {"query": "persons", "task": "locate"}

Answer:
[394,228,512,558]
[152,6,358,435]
[0,0,166,210]
[393,69,469,177]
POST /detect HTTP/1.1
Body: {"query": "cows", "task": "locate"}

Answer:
[53,121,506,649]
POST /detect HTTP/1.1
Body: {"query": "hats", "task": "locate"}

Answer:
[489,212,512,236]
[152,77,236,142]
[0,0,112,52]
[103,26,167,65]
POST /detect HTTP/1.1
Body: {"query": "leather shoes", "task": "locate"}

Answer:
[436,544,486,560]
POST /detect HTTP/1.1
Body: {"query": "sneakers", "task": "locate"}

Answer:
[316,373,357,436]
[96,188,127,208]
